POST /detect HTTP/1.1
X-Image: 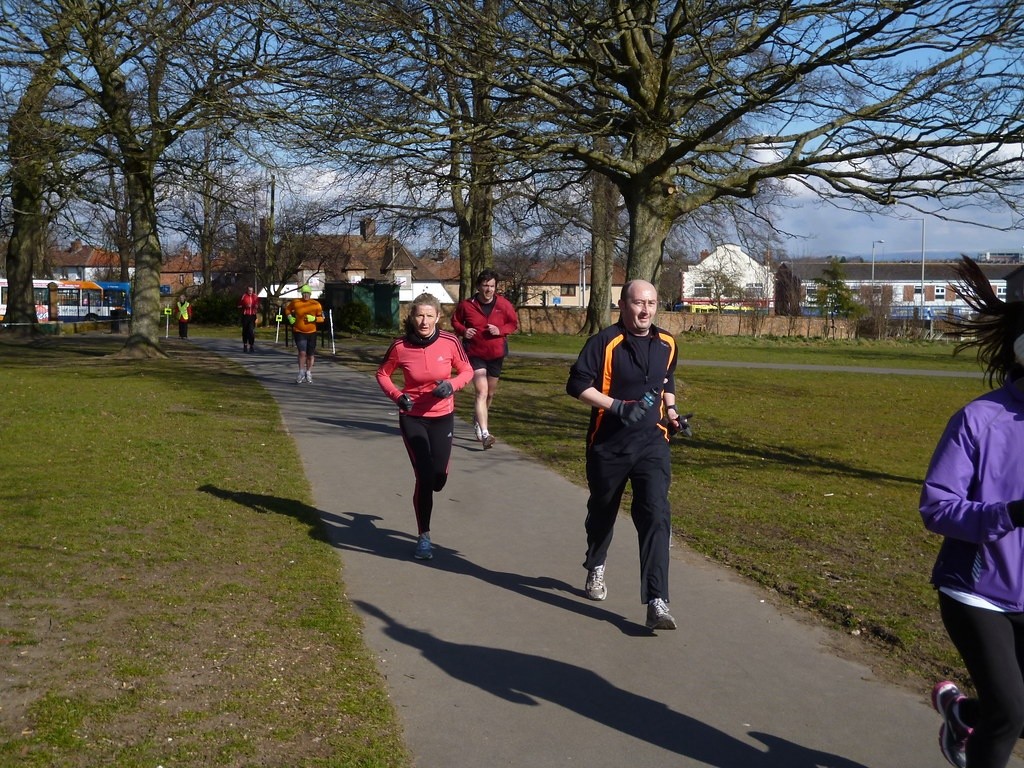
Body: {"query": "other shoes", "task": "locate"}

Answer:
[249,345,254,353]
[244,345,247,352]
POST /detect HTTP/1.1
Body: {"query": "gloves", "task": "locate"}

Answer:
[398,393,414,412]
[303,314,317,324]
[287,314,296,326]
[610,398,648,424]
[656,412,693,443]
[432,380,452,399]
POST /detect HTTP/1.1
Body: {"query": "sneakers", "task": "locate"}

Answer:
[645,598,677,630]
[482,432,495,451]
[473,415,483,442]
[931,681,974,768]
[295,374,305,383]
[584,559,608,601]
[414,533,434,559]
[305,372,312,384]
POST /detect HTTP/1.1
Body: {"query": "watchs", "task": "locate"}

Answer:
[666,404,678,413]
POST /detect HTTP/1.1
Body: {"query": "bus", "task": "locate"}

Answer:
[0,278,133,321]
[680,304,770,317]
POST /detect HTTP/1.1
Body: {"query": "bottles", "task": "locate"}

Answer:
[622,384,660,427]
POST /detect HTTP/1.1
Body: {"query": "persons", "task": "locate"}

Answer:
[375,293,474,559]
[918,254,1024,768]
[174,294,191,339]
[285,284,324,384]
[450,268,518,449]
[240,286,258,353]
[565,280,688,629]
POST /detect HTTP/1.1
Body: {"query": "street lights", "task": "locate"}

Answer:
[899,217,926,326]
[871,239,886,293]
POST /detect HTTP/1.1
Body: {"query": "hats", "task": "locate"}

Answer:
[301,285,312,294]
